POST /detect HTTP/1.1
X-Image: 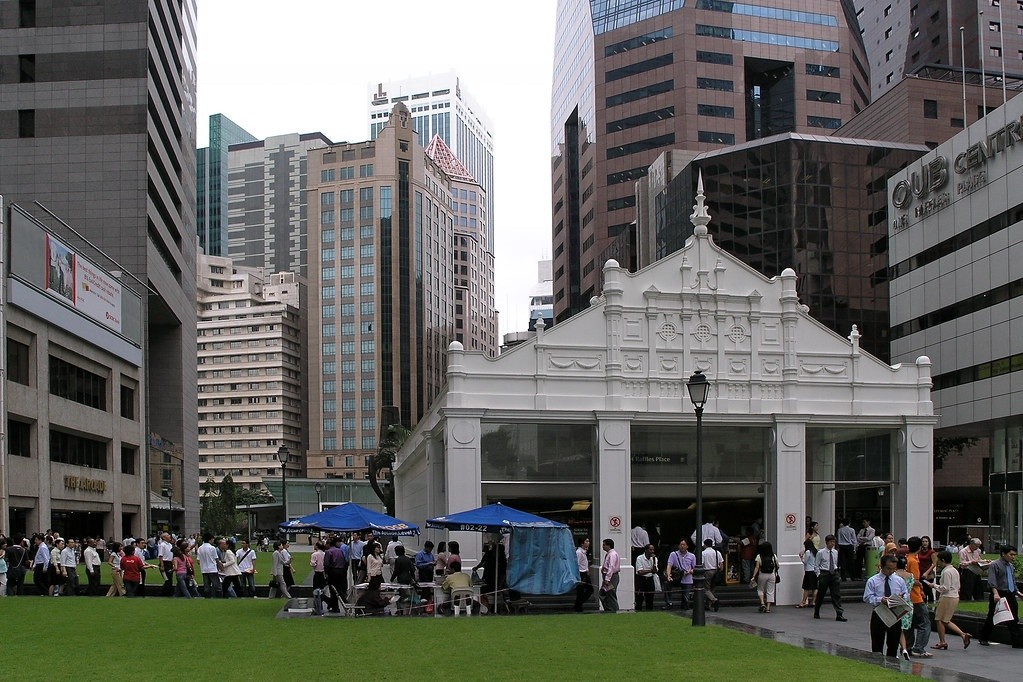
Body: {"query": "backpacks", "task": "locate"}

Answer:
[761,554,774,574]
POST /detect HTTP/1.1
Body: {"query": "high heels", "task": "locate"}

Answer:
[964,633,973,649]
[931,642,948,650]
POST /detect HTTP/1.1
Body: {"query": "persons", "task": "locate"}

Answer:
[436,561,481,616]
[308,531,350,613]
[105,534,160,597]
[575,538,594,613]
[701,539,725,612]
[436,541,447,574]
[83,536,115,562]
[635,544,658,612]
[978,543,1023,647]
[0,528,82,597]
[598,538,620,613]
[447,541,462,569]
[630,520,650,567]
[835,519,991,661]
[195,533,258,598]
[750,542,780,611]
[415,540,437,582]
[54,259,71,295]
[470,543,507,584]
[662,538,694,610]
[794,513,821,608]
[157,533,201,599]
[813,535,848,622]
[740,517,765,584]
[255,534,270,553]
[268,540,296,599]
[83,538,102,596]
[351,531,415,615]
[690,518,729,565]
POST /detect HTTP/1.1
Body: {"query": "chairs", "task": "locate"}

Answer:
[450,589,474,616]
[331,585,373,618]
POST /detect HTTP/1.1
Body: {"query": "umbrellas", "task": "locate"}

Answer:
[425,500,570,533]
[277,499,420,586]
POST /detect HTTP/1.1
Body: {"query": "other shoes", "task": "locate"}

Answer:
[835,615,847,622]
[758,604,766,612]
[813,613,821,619]
[912,651,934,658]
[796,604,807,608]
[663,600,720,612]
[902,650,909,661]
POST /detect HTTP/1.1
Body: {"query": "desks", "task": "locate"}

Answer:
[359,581,444,615]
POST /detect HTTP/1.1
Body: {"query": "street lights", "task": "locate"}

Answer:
[276,444,289,521]
[685,369,712,626]
[315,482,322,541]
[160,486,173,534]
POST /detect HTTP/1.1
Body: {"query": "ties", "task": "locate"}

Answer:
[863,528,867,538]
[1005,563,1014,593]
[829,550,834,574]
[885,576,891,598]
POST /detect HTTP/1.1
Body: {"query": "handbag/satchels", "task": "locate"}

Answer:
[775,571,780,584]
[268,576,281,588]
[664,567,684,585]
[652,573,662,592]
[187,558,194,575]
[711,568,721,586]
[470,569,481,583]
